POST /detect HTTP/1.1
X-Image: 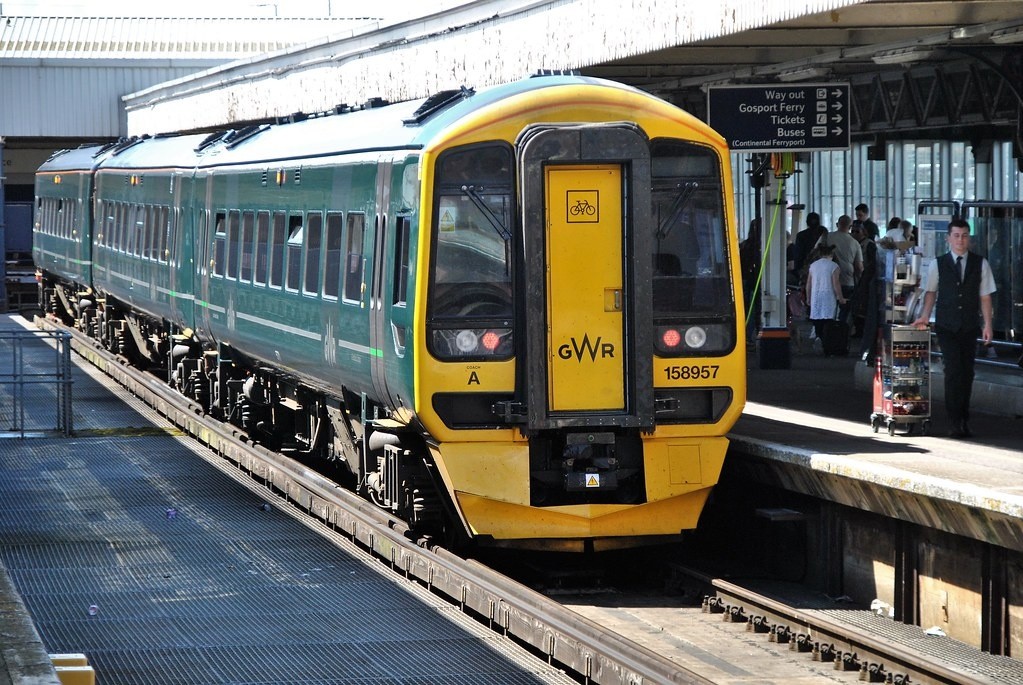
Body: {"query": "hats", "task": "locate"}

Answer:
[854,220,867,234]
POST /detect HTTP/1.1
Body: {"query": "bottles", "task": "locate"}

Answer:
[882,358,929,386]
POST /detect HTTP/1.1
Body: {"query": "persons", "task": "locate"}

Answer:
[784,204,917,362]
[437,221,511,300]
[910,219,997,439]
[739,219,759,350]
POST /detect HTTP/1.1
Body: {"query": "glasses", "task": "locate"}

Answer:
[851,230,864,234]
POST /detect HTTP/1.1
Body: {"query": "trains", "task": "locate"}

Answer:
[34,73,746,553]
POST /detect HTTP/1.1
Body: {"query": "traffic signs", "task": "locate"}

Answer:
[707,82,851,152]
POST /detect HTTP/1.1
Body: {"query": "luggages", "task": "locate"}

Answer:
[824,298,852,357]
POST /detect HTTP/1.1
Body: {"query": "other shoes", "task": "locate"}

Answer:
[948,422,974,438]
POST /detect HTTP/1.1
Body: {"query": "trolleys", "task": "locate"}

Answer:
[870,323,932,437]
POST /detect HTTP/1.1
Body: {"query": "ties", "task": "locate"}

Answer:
[956,255,963,283]
